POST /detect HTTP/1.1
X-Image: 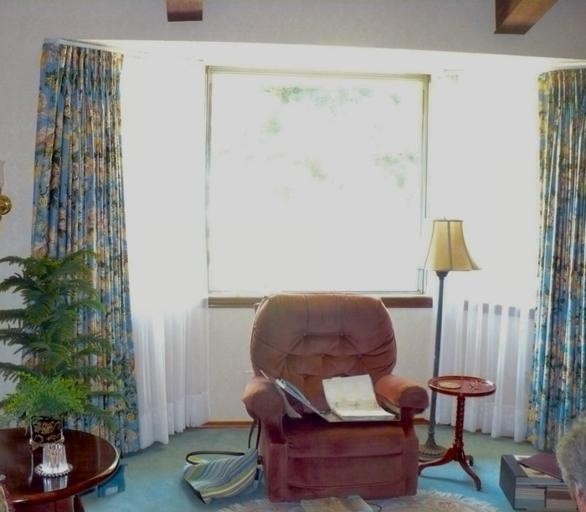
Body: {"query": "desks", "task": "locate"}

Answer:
[416,373,500,493]
[0,426,123,512]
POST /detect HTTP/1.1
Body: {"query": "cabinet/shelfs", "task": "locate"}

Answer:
[498,452,577,510]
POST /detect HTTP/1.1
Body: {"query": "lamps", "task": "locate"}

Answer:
[410,216,486,463]
[0,154,13,218]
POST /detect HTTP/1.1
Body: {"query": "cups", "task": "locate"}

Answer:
[42,478,68,493]
[42,443,68,473]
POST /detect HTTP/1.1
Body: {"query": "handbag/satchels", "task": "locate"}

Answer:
[182,419,261,503]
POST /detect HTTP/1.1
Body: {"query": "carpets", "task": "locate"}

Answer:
[213,493,496,512]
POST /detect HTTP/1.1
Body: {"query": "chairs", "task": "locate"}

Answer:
[243,292,435,503]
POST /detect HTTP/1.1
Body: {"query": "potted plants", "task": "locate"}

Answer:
[0,253,127,479]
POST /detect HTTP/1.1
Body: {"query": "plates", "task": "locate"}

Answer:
[439,382,461,389]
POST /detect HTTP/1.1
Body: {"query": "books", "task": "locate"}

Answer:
[322,373,396,422]
[501,448,578,512]
